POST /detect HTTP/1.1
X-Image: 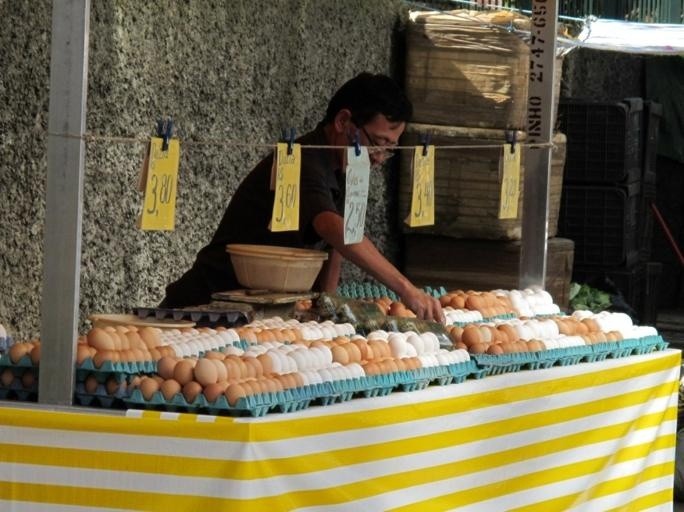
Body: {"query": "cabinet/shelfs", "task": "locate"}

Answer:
[0,348,682,512]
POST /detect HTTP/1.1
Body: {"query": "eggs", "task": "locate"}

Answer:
[77,315,470,406]
[1,339,40,389]
[361,288,659,354]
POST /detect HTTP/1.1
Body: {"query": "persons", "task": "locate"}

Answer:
[159,72,446,324]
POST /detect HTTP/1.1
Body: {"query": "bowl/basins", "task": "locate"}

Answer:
[224,244,329,292]
[91,311,196,333]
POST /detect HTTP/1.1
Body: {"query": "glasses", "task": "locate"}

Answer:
[354,121,395,161]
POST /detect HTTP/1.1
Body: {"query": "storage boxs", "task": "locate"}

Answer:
[393,240,575,320]
[394,9,562,130]
[385,128,568,240]
